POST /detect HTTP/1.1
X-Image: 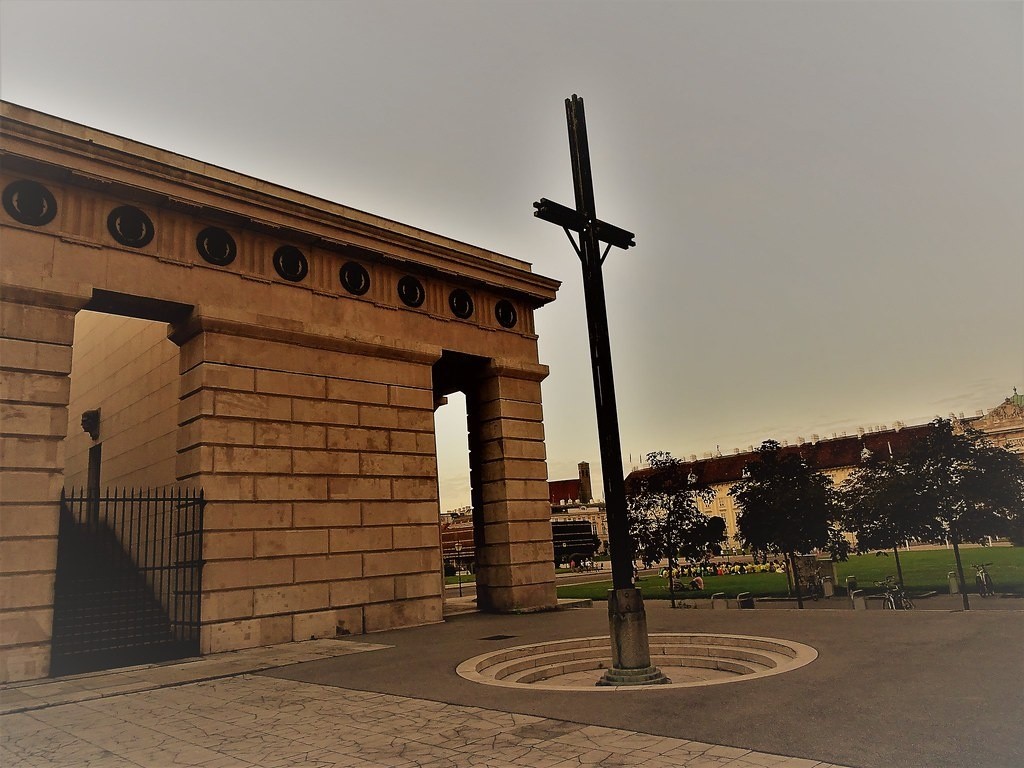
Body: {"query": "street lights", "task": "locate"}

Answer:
[455,540,463,597]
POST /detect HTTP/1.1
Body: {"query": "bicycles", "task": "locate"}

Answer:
[972,563,995,597]
[808,566,825,599]
[873,575,915,610]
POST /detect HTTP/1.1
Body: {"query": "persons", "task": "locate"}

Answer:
[689,573,705,592]
[571,558,599,574]
[658,560,788,579]
[670,572,683,590]
